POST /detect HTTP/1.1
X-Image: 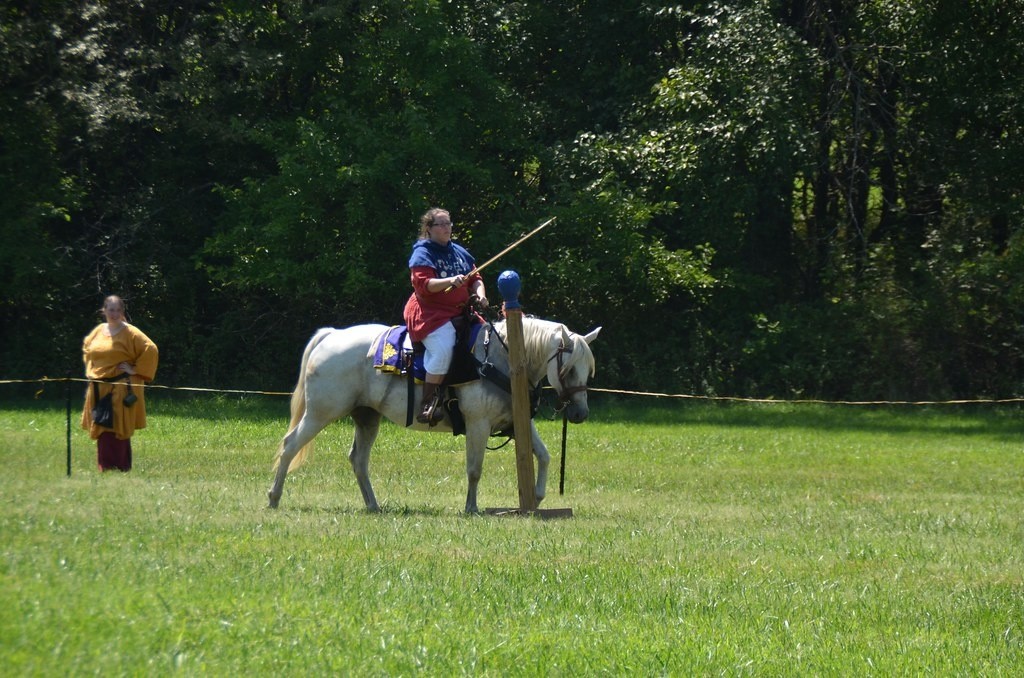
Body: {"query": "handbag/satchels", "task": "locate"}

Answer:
[94,393,113,428]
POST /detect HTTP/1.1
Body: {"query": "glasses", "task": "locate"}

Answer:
[430,222,453,229]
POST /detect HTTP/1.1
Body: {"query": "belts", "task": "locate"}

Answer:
[93,372,129,382]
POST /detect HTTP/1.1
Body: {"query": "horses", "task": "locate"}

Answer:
[266,316,603,517]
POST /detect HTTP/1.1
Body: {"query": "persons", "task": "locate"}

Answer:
[403,209,489,422]
[82,295,159,475]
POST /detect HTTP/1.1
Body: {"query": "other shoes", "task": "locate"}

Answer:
[417,405,443,423]
